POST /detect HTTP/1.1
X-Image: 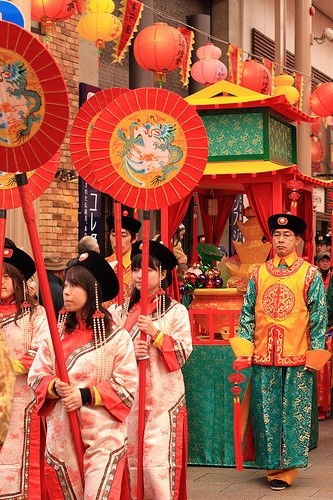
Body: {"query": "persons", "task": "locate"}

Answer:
[3,216,190,322]
[27,251,137,500]
[0,246,49,500]
[314,235,333,329]
[230,214,330,490]
[262,236,270,262]
[106,240,192,500]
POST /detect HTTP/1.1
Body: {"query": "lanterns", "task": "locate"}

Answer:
[31,0,333,168]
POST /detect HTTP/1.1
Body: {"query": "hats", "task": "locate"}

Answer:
[314,234,331,245]
[106,210,141,234]
[3,245,37,351]
[56,250,120,385]
[268,211,307,238]
[317,251,331,261]
[121,240,178,325]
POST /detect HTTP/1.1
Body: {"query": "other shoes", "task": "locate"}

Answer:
[317,415,326,421]
[270,479,287,490]
